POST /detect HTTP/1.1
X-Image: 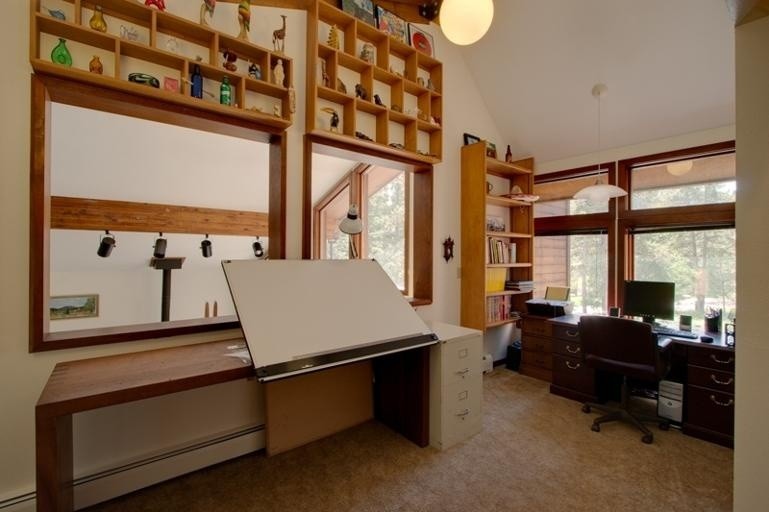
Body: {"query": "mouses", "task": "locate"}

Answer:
[701,336,713,342]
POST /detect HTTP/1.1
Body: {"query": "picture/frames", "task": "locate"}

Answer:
[49,295,99,320]
[408,23,434,60]
[463,132,481,147]
[342,0,375,27]
[376,4,408,46]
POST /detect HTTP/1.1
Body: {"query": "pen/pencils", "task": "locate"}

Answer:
[710,309,720,316]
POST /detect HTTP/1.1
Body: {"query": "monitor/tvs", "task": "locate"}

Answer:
[623,281,675,330]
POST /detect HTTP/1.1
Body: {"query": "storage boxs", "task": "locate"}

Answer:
[487,268,506,291]
[506,345,521,374]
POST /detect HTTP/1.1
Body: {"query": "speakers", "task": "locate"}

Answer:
[610,307,621,317]
[679,315,691,331]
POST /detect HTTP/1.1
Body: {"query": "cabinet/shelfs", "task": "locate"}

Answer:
[427,320,484,452]
[462,138,533,331]
[30,0,294,130]
[306,0,442,172]
[519,313,552,382]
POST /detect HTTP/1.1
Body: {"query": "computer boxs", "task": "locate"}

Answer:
[658,377,685,430]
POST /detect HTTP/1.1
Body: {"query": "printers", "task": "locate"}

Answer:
[525,287,574,316]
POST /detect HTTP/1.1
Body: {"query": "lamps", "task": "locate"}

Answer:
[153,236,166,258]
[573,84,629,206]
[96,232,117,258]
[339,204,364,259]
[666,161,692,175]
[200,237,213,258]
[440,1,494,46]
[252,241,263,257]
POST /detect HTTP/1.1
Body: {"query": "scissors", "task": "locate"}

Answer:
[706,305,718,313]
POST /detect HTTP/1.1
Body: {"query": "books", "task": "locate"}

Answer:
[505,280,535,291]
[486,294,511,323]
[486,236,516,264]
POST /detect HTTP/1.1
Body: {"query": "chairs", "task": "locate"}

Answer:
[578,316,671,443]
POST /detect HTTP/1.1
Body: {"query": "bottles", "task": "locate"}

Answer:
[220,75,231,106]
[191,64,204,98]
[505,144,513,164]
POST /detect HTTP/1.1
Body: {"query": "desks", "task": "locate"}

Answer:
[34,334,440,512]
[548,315,735,452]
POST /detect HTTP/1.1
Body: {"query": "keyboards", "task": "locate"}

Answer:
[653,327,698,339]
